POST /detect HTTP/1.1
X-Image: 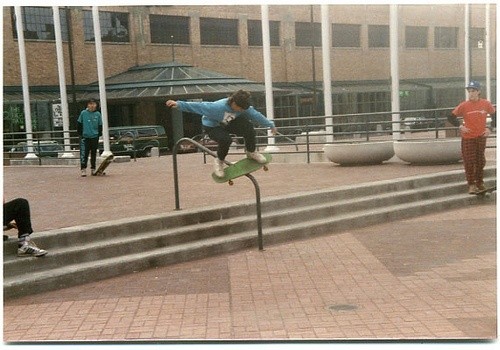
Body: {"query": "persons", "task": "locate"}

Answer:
[76,98,102,176]
[447,81,496,194]
[3,198,48,256]
[166,90,277,177]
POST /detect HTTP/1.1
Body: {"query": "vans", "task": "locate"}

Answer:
[97,125,170,157]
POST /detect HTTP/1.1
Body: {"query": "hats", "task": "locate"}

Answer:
[465,81,482,91]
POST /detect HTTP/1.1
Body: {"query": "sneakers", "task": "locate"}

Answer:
[82,168,86,177]
[469,184,476,194]
[479,184,486,190]
[215,159,225,178]
[247,151,267,163]
[18,244,47,257]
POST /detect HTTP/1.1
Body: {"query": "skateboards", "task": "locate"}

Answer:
[212,151,272,185]
[476,186,496,197]
[92,154,113,176]
